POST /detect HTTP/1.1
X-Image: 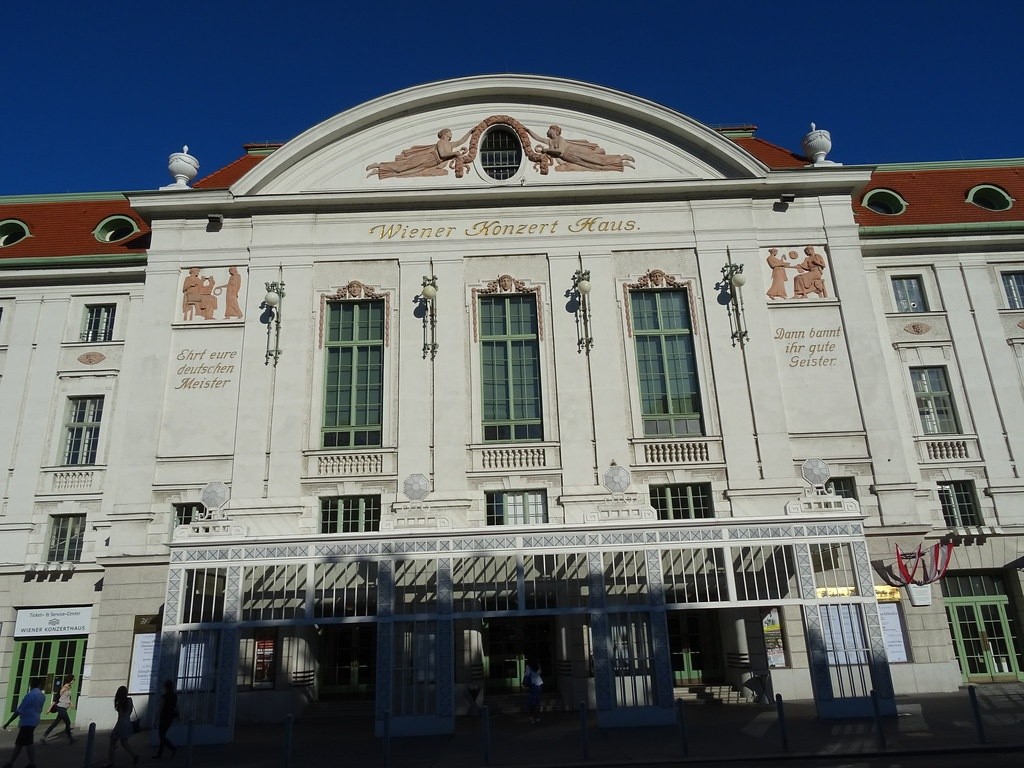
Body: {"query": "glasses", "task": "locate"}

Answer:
[72,679,75,681]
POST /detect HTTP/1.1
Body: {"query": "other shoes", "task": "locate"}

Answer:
[131,755,139,767]
[69,738,79,745]
[152,755,161,759]
[101,762,113,768]
[25,764,35,768]
[170,748,178,758]
[40,736,49,745]
[3,762,12,768]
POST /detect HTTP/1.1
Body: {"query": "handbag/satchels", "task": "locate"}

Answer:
[130,719,142,734]
[49,698,59,713]
[522,676,530,687]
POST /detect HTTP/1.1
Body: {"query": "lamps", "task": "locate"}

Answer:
[35,562,49,573]
[60,561,76,573]
[47,561,61,573]
[990,525,1003,534]
[23,563,36,573]
[421,275,439,356]
[723,262,750,343]
[977,525,992,535]
[965,526,980,535]
[263,280,286,365]
[953,527,967,537]
[574,269,594,350]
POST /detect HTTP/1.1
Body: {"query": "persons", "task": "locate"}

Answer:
[40,675,79,746]
[650,270,666,288]
[3,678,46,768]
[499,275,512,293]
[183,266,243,320]
[349,282,364,298]
[521,123,635,173]
[525,654,543,724]
[101,686,139,768]
[912,323,922,335]
[366,126,477,179]
[766,246,828,300]
[86,352,97,364]
[152,681,180,759]
